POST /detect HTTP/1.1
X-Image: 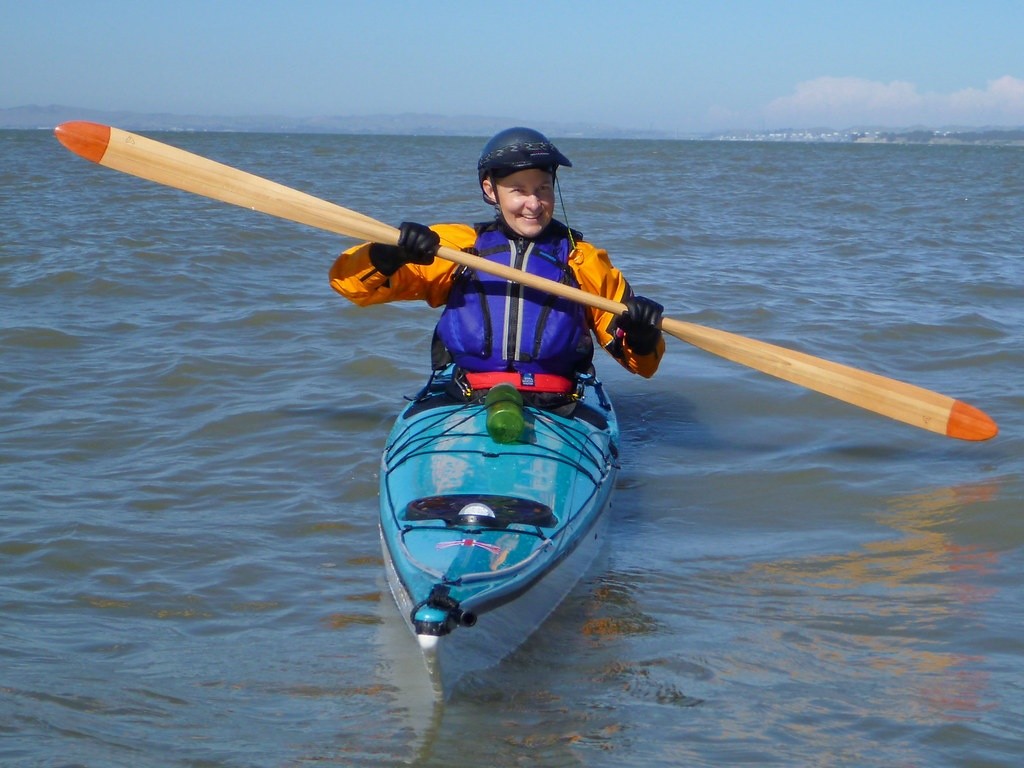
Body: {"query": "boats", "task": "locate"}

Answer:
[375,352,620,693]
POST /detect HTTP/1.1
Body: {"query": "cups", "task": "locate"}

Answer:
[486,382,525,443]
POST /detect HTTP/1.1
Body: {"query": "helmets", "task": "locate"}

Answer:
[476,126,572,194]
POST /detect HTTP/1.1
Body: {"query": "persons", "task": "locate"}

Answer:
[329,127,665,411]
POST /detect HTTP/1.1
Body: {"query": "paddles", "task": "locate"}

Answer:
[52,118,1000,445]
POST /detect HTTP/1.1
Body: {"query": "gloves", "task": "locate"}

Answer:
[615,296,664,356]
[369,222,439,277]
